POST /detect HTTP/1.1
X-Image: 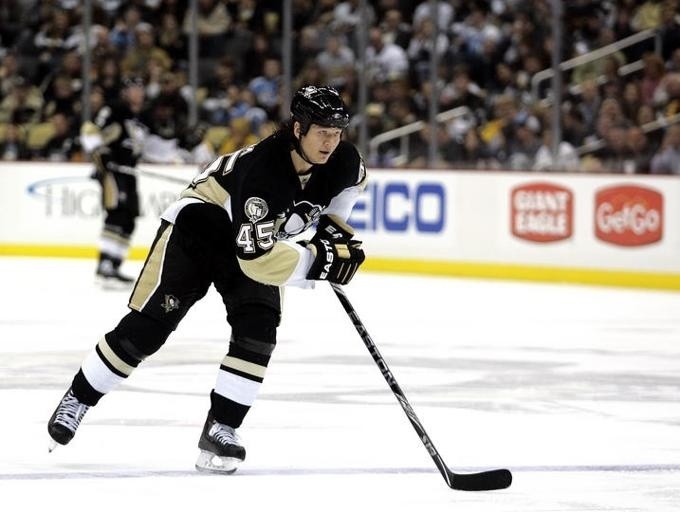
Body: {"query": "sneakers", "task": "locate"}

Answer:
[48,384,89,444]
[198,416,251,460]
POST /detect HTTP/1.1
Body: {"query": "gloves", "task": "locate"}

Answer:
[298,216,365,285]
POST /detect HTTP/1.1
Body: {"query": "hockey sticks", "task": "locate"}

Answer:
[325,277,512,491]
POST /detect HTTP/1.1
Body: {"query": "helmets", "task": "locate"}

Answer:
[290,83,350,128]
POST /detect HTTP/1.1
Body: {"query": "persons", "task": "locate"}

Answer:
[79,73,209,288]
[48,84,367,462]
[0,1,679,177]
